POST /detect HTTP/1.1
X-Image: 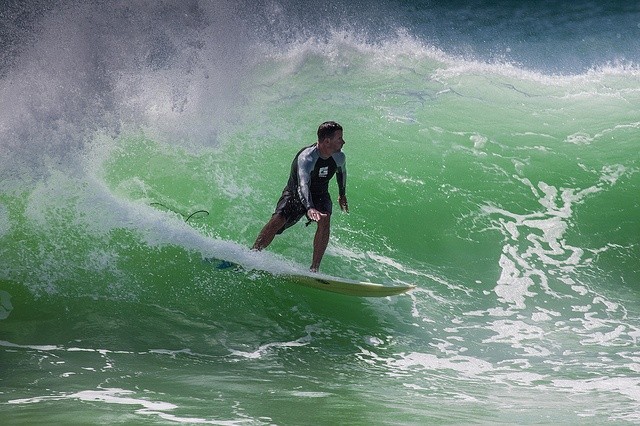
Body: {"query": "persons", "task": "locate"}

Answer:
[249,122,351,277]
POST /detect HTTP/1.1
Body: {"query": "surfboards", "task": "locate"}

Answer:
[288,272,414,298]
